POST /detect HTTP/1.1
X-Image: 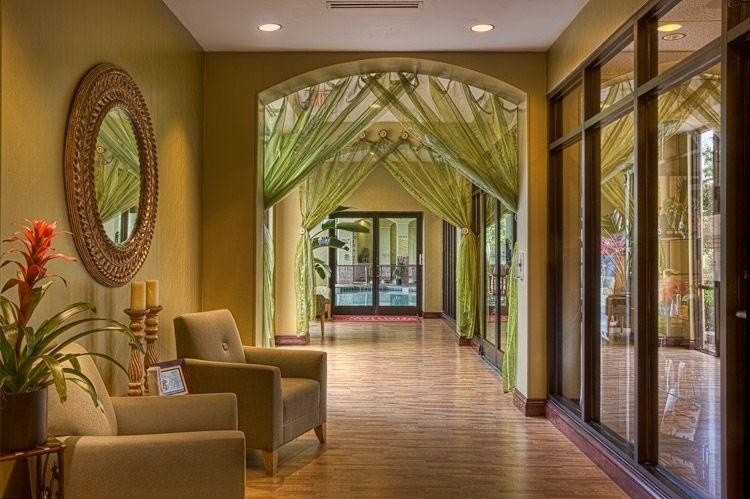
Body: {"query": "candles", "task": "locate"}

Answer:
[131,282,146,310]
[146,280,159,307]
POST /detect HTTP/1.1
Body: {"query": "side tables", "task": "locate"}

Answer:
[0,432,68,499]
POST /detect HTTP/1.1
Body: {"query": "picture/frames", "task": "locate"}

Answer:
[151,360,194,394]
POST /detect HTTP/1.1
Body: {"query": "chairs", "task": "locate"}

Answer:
[316,295,326,339]
[174,309,326,476]
[28,343,247,499]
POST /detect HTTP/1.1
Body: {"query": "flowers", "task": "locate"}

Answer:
[0,219,145,413]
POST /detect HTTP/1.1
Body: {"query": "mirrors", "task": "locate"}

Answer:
[63,63,159,288]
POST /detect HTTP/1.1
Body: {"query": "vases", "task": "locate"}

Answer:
[0,385,47,451]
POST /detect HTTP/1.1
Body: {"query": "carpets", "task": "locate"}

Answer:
[331,315,421,323]
[489,316,507,323]
